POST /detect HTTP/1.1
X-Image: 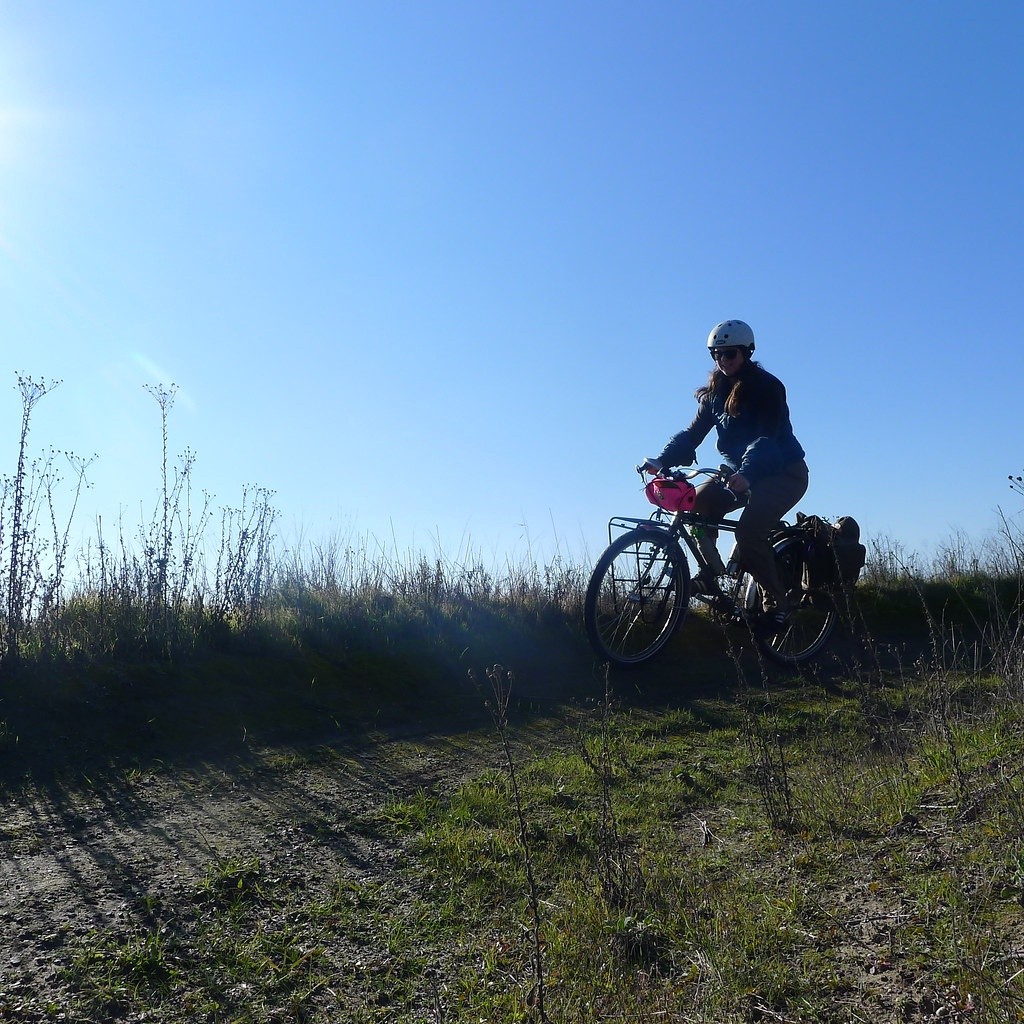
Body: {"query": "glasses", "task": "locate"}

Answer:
[710,349,741,359]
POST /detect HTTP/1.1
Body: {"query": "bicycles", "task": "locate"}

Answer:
[581,456,867,670]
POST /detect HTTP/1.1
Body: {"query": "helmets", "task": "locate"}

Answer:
[707,319,755,352]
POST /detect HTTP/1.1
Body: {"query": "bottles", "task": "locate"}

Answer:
[692,528,726,577]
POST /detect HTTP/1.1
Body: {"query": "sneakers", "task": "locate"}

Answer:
[766,606,785,630]
[689,578,718,595]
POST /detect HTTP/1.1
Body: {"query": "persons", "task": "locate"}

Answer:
[647,320,809,630]
